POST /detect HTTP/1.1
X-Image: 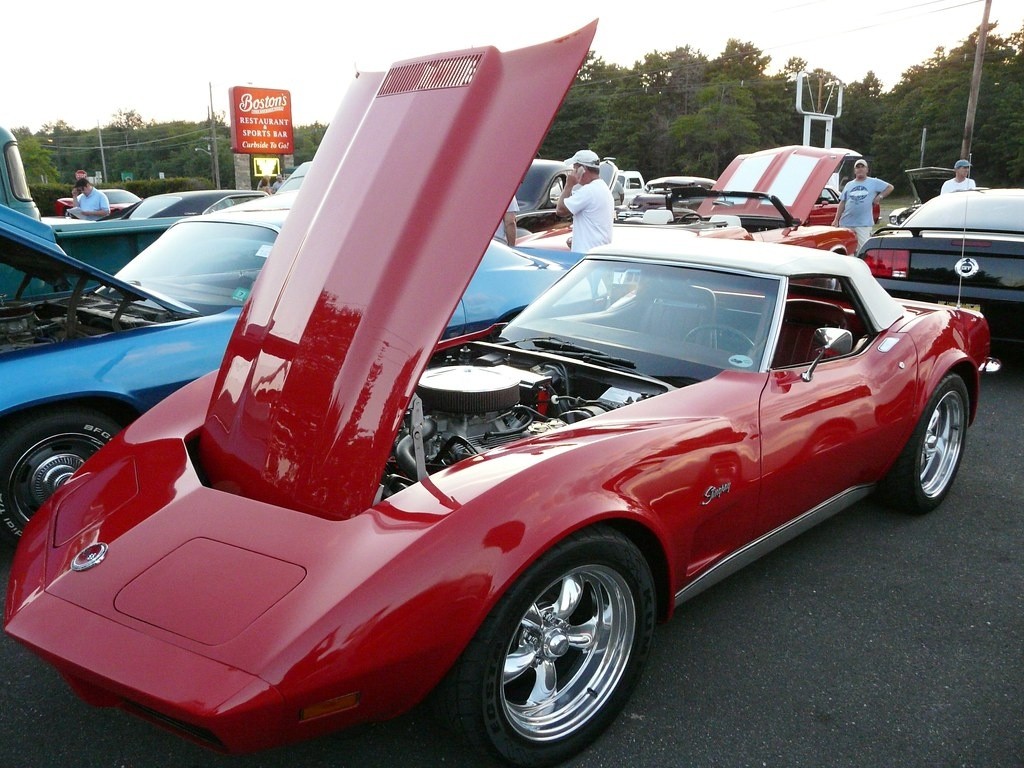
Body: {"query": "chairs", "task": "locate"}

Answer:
[638,283,719,350]
[708,214,742,228]
[643,208,675,223]
[769,298,847,367]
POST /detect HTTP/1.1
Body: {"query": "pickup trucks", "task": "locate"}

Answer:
[1,125,195,296]
[55,189,139,218]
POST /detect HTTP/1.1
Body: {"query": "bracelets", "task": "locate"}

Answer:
[879,193,883,199]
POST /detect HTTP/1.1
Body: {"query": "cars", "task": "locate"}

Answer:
[857,189,1024,356]
[4,18,989,768]
[628,175,716,210]
[806,186,881,223]
[887,166,956,226]
[505,158,621,240]
[515,144,863,259]
[0,194,586,550]
[108,188,267,224]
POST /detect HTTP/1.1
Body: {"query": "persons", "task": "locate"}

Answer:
[494,195,520,246]
[556,150,614,253]
[72,179,111,220]
[831,159,894,253]
[257,174,287,195]
[940,159,976,194]
[612,180,625,206]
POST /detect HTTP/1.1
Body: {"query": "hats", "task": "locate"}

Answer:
[854,159,867,168]
[564,150,600,168]
[954,160,973,169]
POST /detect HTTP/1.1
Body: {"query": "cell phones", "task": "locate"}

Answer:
[577,166,586,177]
